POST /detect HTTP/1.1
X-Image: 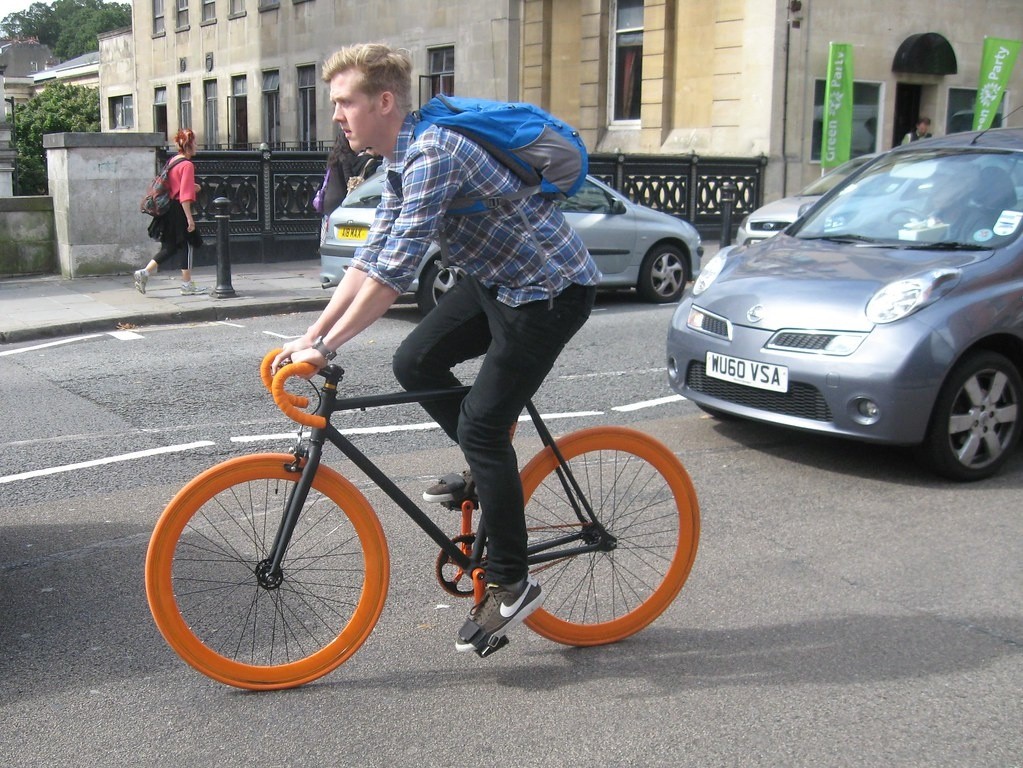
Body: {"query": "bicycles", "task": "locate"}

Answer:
[143,340,704,692]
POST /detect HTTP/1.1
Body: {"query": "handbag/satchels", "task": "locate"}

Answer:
[313,189,325,215]
[346,158,375,197]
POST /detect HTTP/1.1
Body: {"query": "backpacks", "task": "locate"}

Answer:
[419,93,588,219]
[140,155,189,217]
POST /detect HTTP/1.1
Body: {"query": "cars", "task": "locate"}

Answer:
[665,123,1023,482]
[318,159,704,322]
[735,149,1017,269]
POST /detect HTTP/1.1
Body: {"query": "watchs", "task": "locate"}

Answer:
[312,335,337,361]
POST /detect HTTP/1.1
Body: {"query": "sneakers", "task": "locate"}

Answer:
[134,269,148,294]
[455,574,544,651]
[181,281,206,295]
[422,470,478,502]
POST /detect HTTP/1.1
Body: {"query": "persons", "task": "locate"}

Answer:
[133,128,210,297]
[900,116,934,148]
[352,147,381,183]
[269,40,604,654]
[323,128,357,218]
[864,117,877,152]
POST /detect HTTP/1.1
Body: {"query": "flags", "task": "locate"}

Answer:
[819,41,854,167]
[972,36,1022,132]
